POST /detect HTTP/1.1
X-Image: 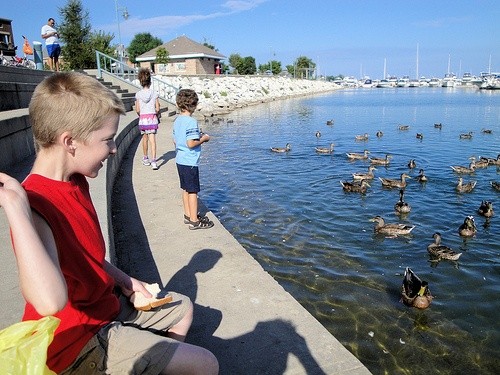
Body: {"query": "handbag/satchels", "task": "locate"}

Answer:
[23,38,33,55]
[0,315,61,375]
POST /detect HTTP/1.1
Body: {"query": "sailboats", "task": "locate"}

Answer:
[377,57,395,88]
[442,55,462,87]
[480,56,500,89]
[408,43,421,87]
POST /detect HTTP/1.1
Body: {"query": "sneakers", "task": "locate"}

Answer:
[151,162,158,170]
[141,159,150,166]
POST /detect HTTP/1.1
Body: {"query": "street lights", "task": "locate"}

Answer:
[116,7,131,78]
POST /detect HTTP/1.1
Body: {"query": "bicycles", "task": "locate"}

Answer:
[10,35,38,70]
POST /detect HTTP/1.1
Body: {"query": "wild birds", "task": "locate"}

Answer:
[458,215,476,236]
[316,120,493,140]
[340,150,426,238]
[315,143,334,152]
[271,143,291,152]
[427,232,463,261]
[401,266,433,308]
[478,200,494,217]
[449,155,500,192]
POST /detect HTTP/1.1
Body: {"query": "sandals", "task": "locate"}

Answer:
[189,220,214,230]
[184,214,209,224]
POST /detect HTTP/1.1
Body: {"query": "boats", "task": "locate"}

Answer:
[462,72,489,81]
[332,77,379,88]
[419,76,442,87]
[391,75,409,87]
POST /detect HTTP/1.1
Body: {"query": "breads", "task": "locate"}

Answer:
[134,282,173,311]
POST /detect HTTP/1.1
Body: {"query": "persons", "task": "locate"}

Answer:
[41,18,61,72]
[0,70,218,375]
[135,68,160,170]
[171,89,213,230]
